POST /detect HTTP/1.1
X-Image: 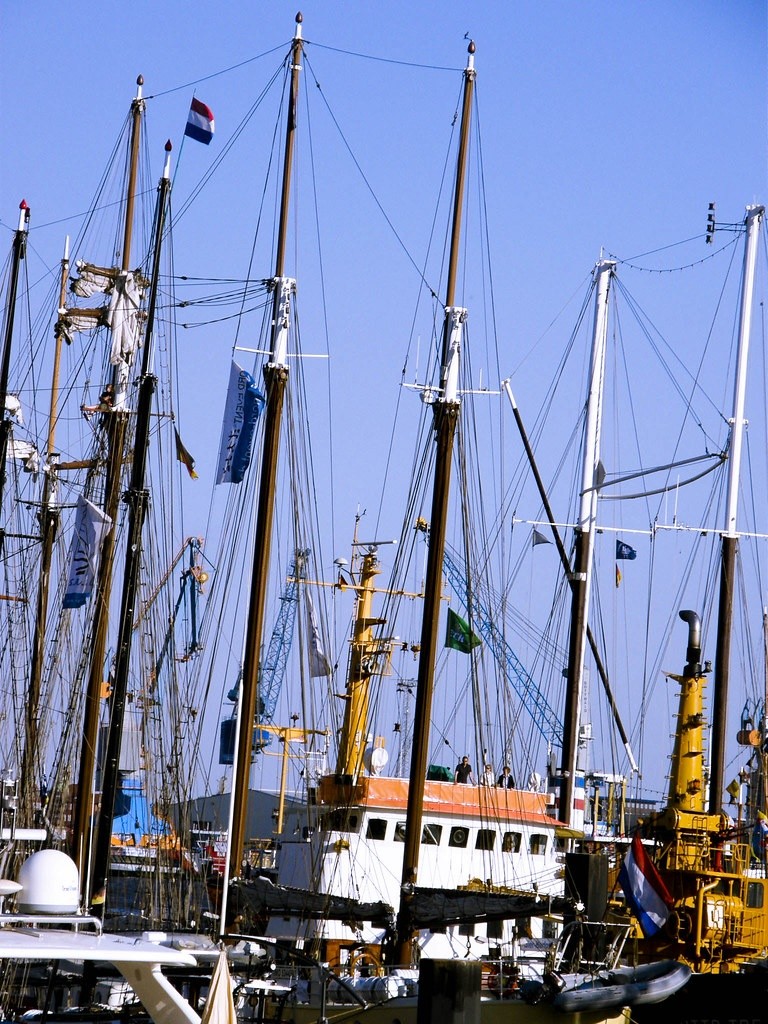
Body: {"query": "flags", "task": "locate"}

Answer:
[173,425,199,480]
[615,539,637,560]
[214,359,265,486]
[445,607,482,654]
[184,97,214,145]
[616,830,674,939]
[305,586,331,678]
[616,562,622,588]
[532,530,553,547]
[62,493,113,608]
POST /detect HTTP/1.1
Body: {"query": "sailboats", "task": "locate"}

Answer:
[0,11,767,1024]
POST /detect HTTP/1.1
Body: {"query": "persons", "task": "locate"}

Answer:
[454,756,476,786]
[479,765,495,787]
[496,766,514,788]
